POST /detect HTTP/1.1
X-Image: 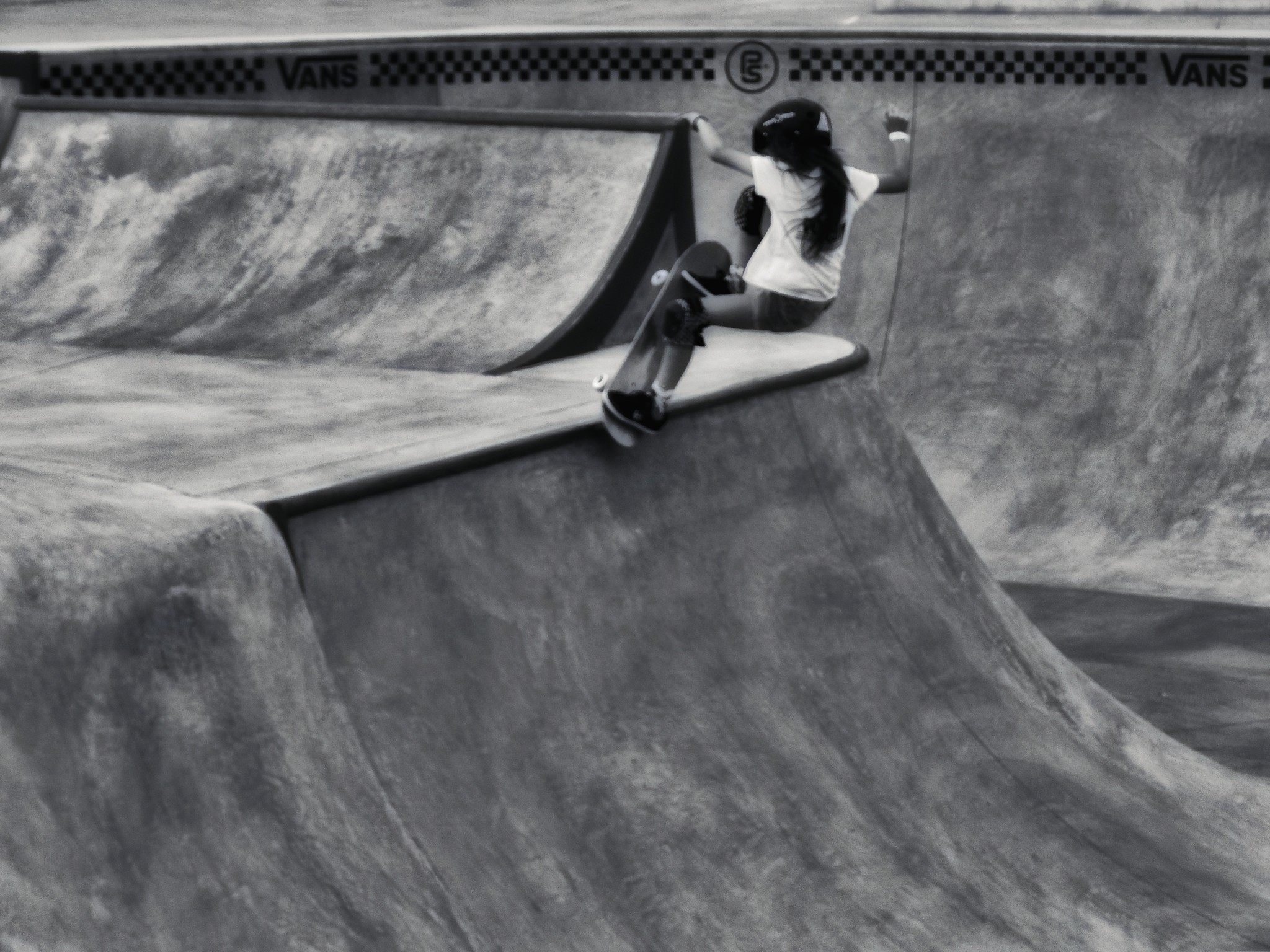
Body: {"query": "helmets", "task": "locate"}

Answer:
[751,97,833,160]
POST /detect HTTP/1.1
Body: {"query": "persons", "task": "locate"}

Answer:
[600,97,900,436]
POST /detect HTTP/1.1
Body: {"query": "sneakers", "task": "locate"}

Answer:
[679,264,747,297]
[603,389,673,436]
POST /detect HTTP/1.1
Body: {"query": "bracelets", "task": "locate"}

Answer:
[693,114,710,131]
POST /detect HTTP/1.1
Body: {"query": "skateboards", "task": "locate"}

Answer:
[587,236,734,452]
